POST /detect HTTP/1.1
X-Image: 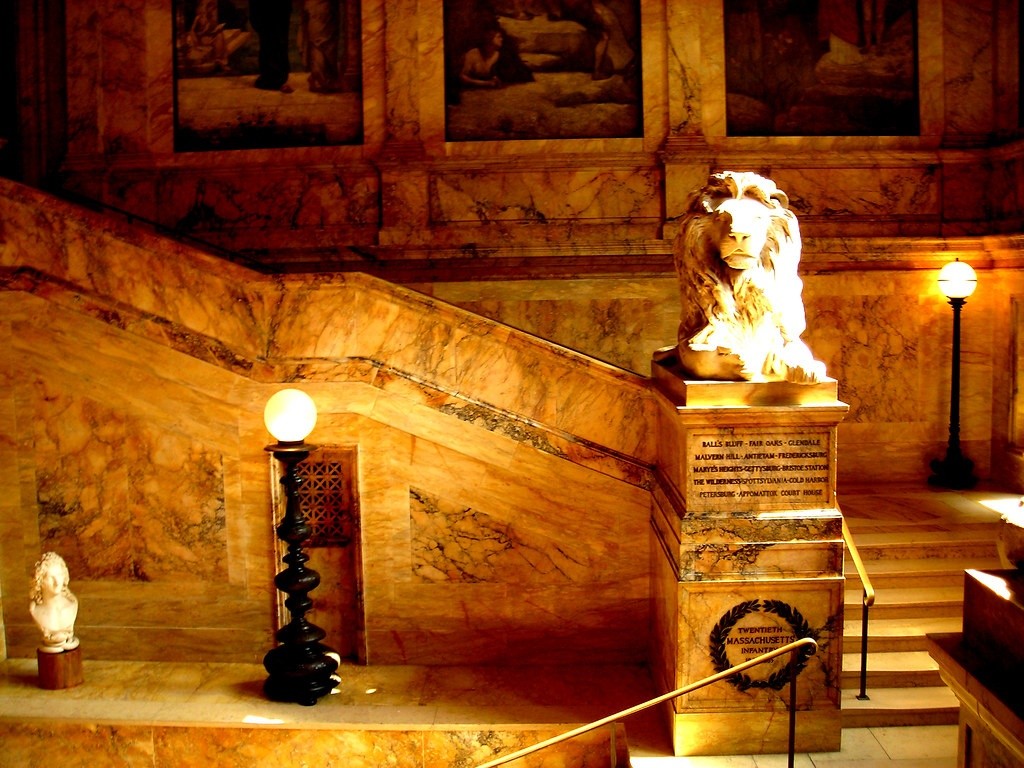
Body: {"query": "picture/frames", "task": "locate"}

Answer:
[145,0,388,162]
[415,0,670,157]
[698,0,947,154]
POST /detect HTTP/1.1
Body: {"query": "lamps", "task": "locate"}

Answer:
[931,260,982,493]
[259,384,350,705]
